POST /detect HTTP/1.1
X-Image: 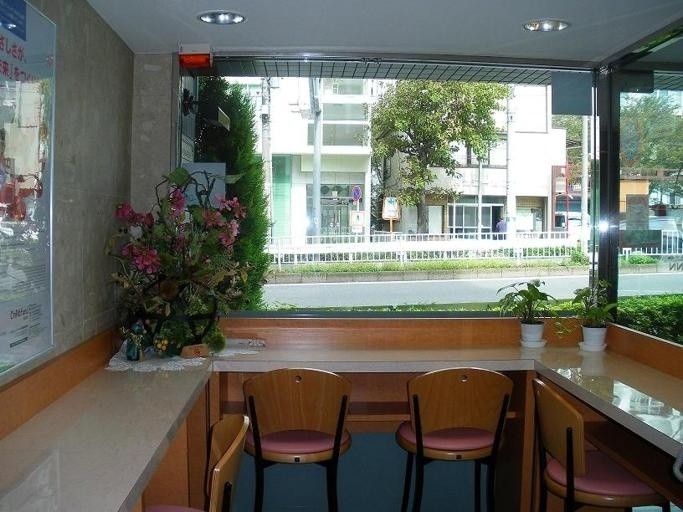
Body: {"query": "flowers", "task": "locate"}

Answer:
[107,167,252,358]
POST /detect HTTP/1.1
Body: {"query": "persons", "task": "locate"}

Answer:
[496,216,507,238]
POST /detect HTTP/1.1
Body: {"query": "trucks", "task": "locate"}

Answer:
[618,217,682,254]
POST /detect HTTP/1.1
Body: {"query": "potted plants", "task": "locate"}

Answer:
[571,277,617,351]
[484,275,563,348]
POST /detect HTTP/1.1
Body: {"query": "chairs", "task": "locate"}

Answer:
[195,411,252,512]
[243,365,352,512]
[392,363,513,512]
[529,377,672,512]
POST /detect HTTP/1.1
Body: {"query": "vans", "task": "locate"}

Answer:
[555,212,590,241]
